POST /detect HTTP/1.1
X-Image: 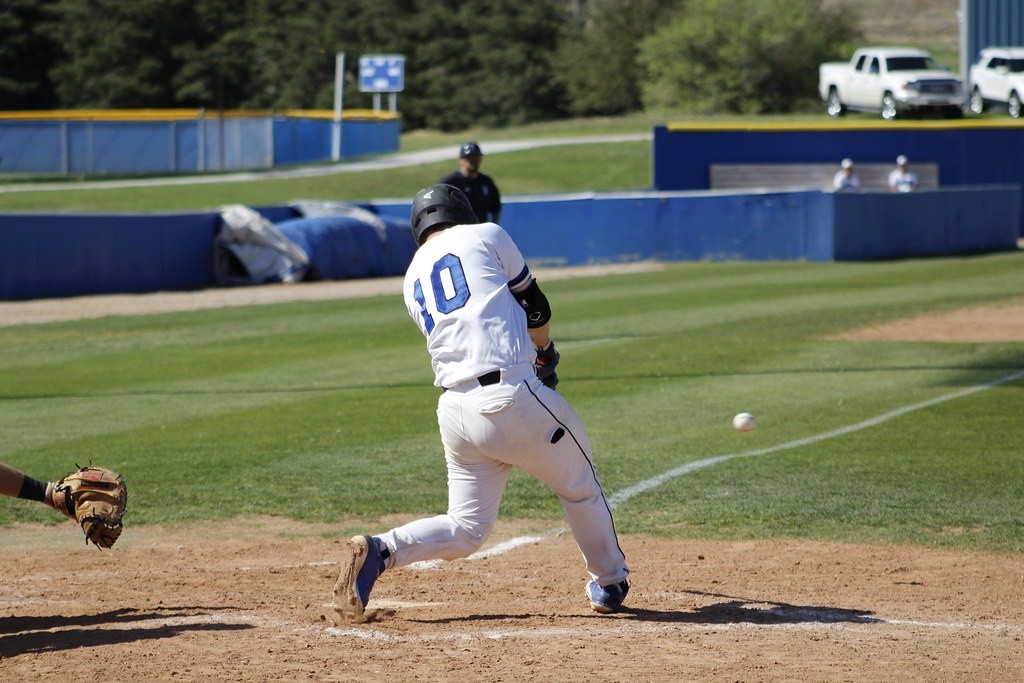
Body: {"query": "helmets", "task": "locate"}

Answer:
[410,183,480,249]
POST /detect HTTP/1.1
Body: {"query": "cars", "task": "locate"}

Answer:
[969,46,1024,118]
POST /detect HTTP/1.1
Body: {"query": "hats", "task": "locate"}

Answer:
[460,142,484,160]
[841,158,854,170]
[896,155,909,166]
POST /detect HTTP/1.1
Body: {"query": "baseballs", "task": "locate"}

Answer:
[732,412,755,430]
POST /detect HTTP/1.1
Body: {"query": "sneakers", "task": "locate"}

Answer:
[586,576,632,614]
[331,535,390,618]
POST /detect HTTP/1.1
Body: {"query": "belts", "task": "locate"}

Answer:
[440,371,500,393]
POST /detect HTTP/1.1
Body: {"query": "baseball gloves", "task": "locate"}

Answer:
[44,467,127,551]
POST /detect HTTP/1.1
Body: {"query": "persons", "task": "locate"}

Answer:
[888,155,919,192]
[0,458,127,548]
[440,143,501,223]
[834,158,861,190]
[332,184,632,620]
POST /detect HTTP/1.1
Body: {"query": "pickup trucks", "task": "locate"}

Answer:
[819,45,965,121]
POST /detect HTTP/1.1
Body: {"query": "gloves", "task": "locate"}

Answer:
[532,341,560,391]
